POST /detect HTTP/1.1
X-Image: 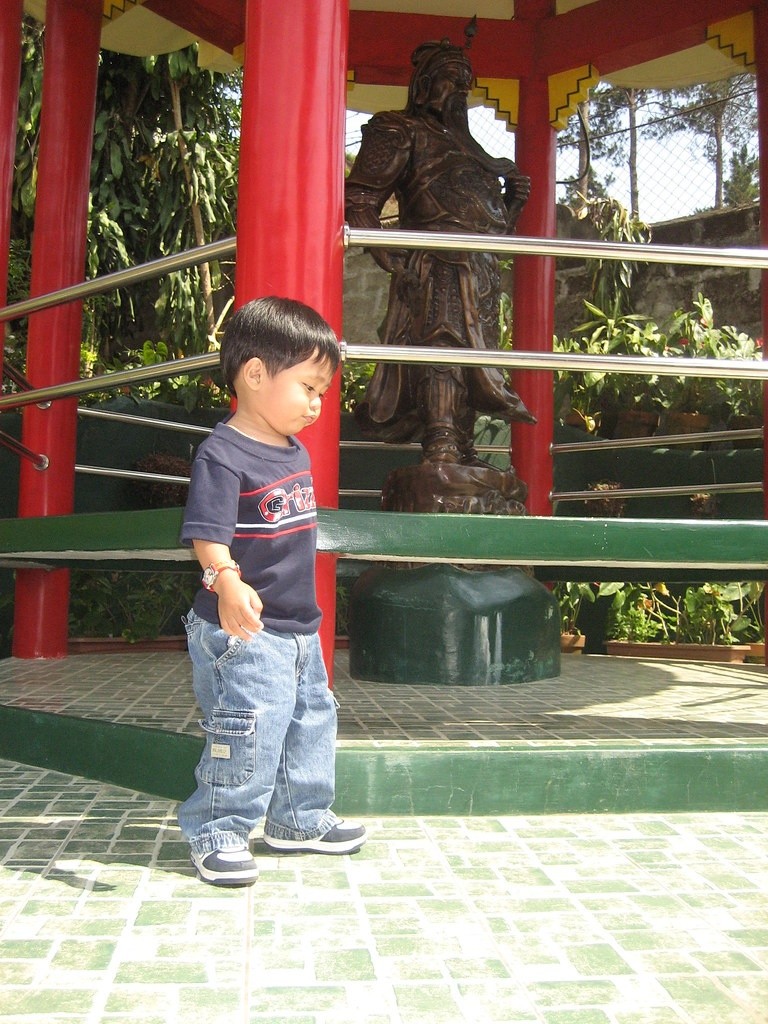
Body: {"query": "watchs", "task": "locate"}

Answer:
[201,559,241,593]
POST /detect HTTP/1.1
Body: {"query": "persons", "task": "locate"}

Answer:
[178,294,370,890]
[343,14,537,477]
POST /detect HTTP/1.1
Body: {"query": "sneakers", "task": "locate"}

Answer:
[190,843,259,885]
[264,817,367,854]
